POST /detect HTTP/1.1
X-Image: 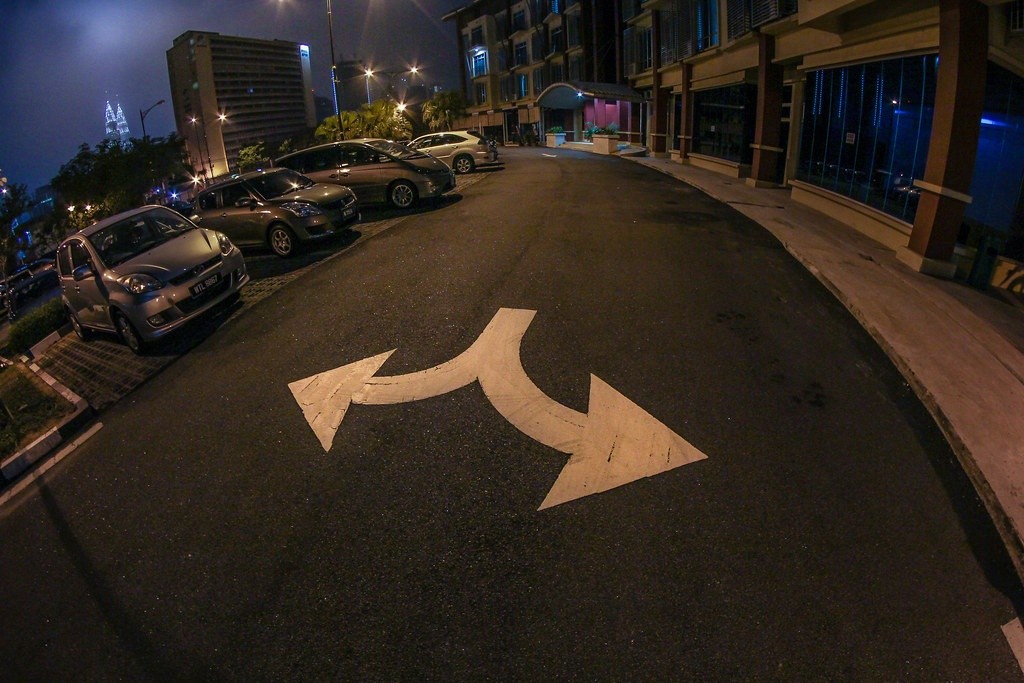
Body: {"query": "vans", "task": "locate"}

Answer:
[274,136,457,210]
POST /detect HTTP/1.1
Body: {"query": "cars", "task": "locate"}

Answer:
[56,204,253,356]
[187,166,365,259]
[405,130,499,173]
